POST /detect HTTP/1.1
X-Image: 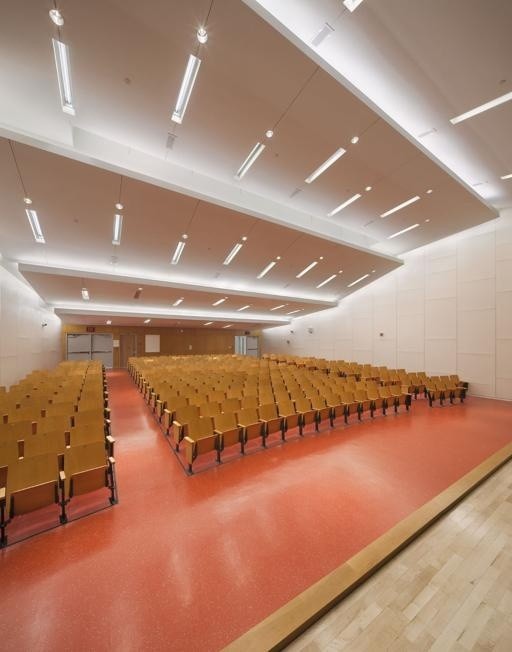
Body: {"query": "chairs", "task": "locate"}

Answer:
[125,352,472,477]
[0,355,119,548]
